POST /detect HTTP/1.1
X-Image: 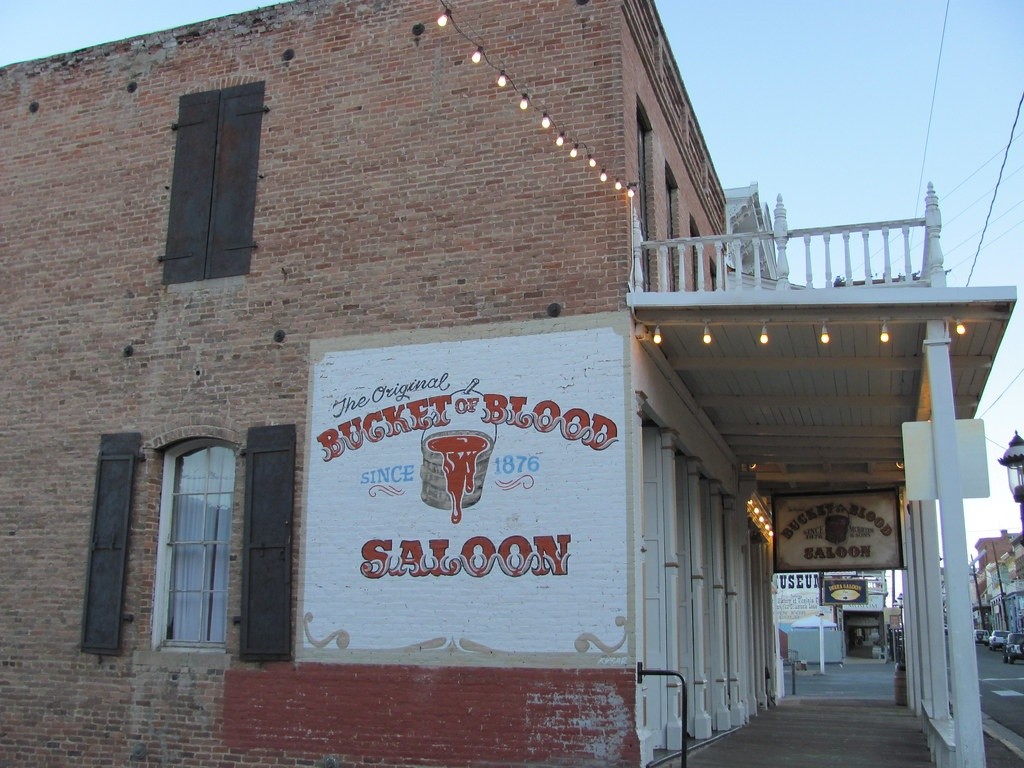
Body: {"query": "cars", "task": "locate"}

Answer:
[988,630,1012,651]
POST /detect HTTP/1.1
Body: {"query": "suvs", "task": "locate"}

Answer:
[1003,633,1024,664]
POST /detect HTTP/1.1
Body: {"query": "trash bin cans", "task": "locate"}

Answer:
[893,662,906,706]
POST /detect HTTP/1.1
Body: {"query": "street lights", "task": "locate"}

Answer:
[897,592,904,664]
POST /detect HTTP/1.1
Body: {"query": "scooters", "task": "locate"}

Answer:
[974,630,989,646]
